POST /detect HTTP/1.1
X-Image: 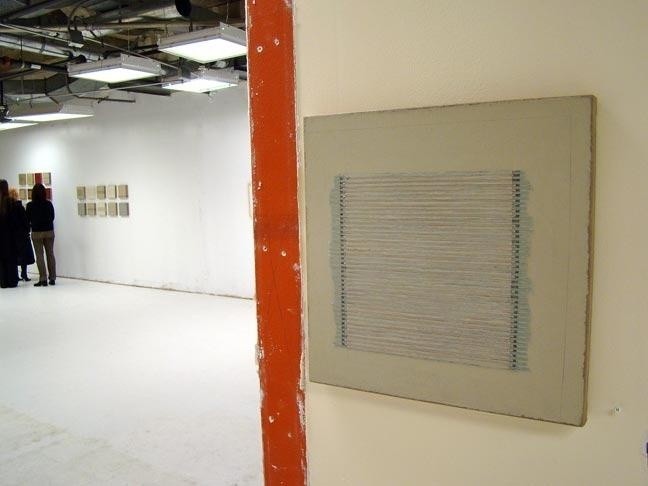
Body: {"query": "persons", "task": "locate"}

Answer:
[8,188,35,281]
[0,179,20,287]
[25,183,56,287]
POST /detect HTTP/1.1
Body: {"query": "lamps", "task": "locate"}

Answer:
[66,21,246,95]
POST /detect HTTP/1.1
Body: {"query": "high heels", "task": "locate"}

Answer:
[21,273,31,281]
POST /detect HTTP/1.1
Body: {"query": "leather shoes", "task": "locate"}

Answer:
[49,280,55,285]
[34,280,47,286]
